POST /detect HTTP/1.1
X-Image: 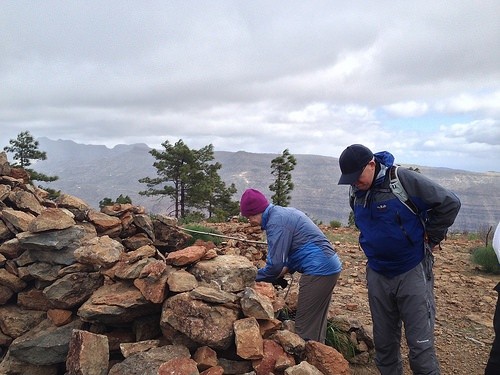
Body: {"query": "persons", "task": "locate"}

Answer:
[338,144,462,375]
[240,189,342,344]
[485,220,500,375]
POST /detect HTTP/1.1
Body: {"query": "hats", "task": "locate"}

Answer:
[338,144,373,185]
[239,188,270,217]
